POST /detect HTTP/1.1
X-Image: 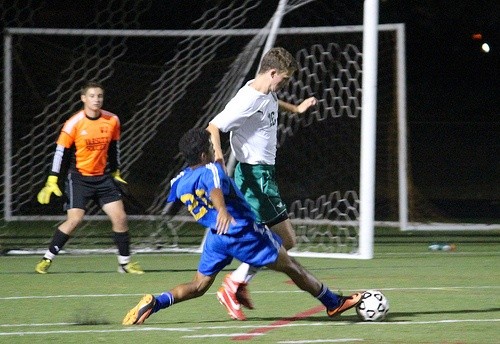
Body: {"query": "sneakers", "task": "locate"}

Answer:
[36,258,53,274]
[224,274,253,309]
[118,261,145,275]
[122,294,157,325]
[217,277,245,318]
[327,289,362,317]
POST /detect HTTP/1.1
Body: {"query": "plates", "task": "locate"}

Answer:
[428,245,451,252]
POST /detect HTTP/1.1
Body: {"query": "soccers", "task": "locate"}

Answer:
[356,289,389,322]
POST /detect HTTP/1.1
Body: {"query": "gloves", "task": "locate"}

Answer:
[37,175,62,204]
[111,169,128,184]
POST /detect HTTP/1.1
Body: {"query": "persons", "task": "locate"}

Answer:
[36,83,146,274]
[122,128,362,325]
[208,48,319,321]
